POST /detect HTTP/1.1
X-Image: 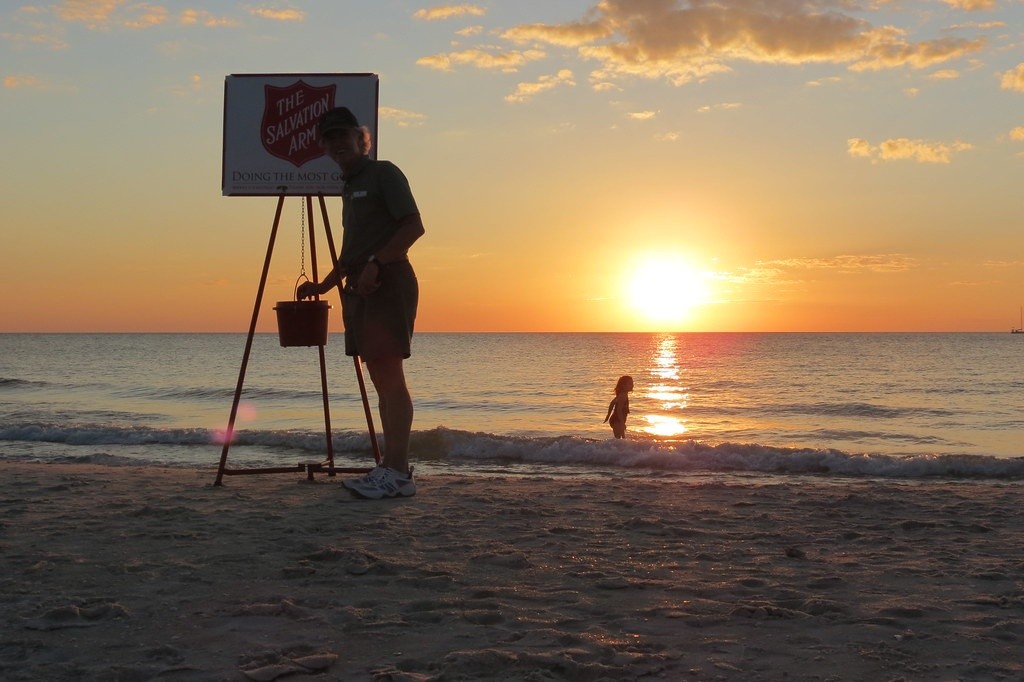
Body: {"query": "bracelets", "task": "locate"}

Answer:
[368,255,382,269]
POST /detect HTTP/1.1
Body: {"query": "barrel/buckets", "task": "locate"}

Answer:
[272,300,332,348]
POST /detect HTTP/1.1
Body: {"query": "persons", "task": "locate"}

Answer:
[296,107,425,501]
[603,376,634,439]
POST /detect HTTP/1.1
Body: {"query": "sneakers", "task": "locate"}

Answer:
[342,464,384,492]
[350,466,416,499]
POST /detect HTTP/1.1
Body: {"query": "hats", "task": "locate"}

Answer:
[319,106,359,136]
